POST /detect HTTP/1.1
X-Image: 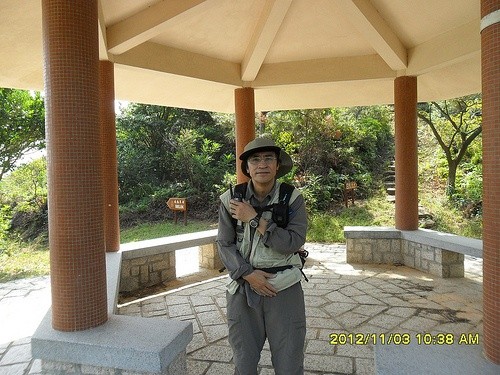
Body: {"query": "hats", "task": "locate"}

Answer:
[238,138,281,160]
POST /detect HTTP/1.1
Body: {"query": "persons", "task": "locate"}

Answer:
[217,135,309,375]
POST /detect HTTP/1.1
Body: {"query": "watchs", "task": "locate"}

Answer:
[249,214,262,228]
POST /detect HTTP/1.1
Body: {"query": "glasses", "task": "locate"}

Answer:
[247,157,277,164]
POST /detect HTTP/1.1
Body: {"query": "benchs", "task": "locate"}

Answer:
[30,313,193,375]
[400,227,483,278]
[342,224,401,268]
[373,337,500,375]
[119,229,225,295]
[106,252,124,313]
